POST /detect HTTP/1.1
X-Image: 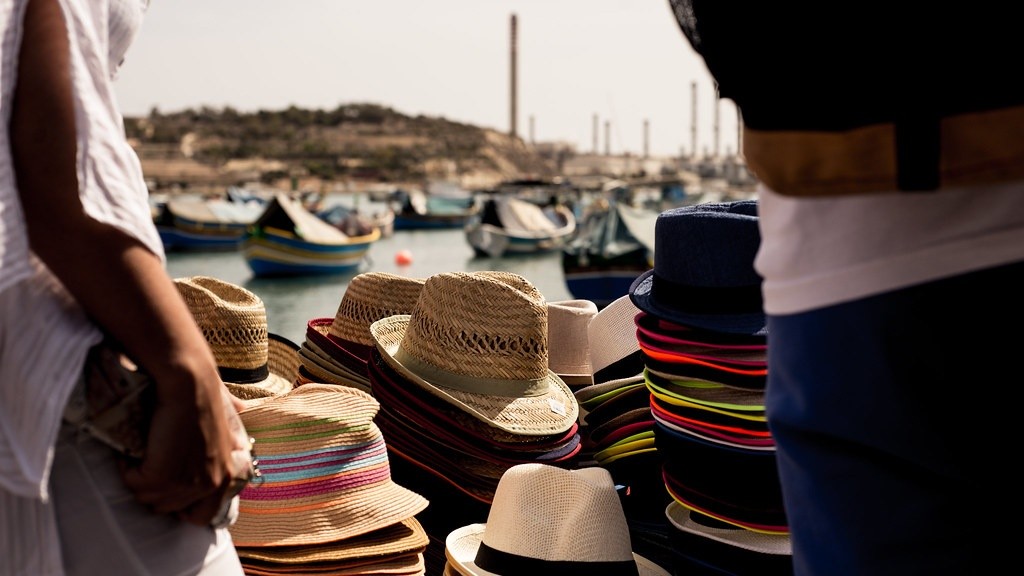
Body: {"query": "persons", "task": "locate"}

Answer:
[682,0,1024,576]
[0,0,255,576]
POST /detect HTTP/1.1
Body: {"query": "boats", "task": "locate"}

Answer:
[145,168,760,251]
[165,193,266,248]
[461,194,577,257]
[239,190,381,281]
[563,201,654,309]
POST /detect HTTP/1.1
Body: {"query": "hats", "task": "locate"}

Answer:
[84,201,794,575]
